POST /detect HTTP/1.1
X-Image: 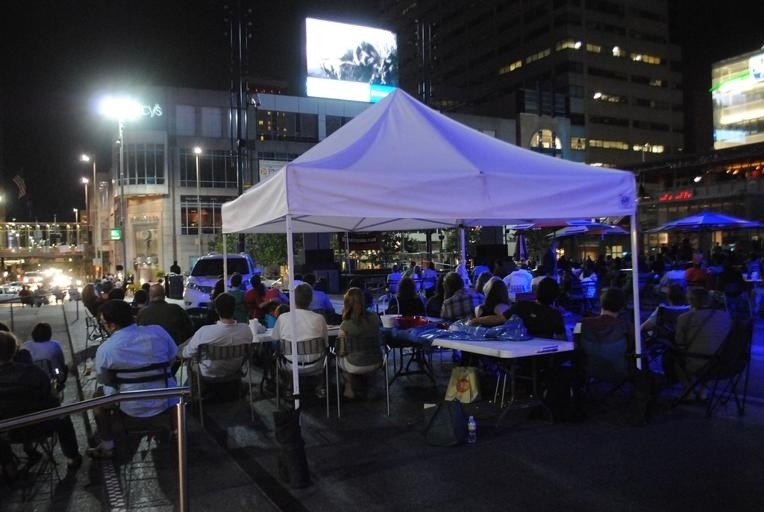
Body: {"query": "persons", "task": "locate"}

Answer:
[2,238,764,466]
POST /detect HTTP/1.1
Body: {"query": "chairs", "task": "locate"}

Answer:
[1,247,764,511]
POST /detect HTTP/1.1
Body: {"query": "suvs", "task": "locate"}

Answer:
[182,251,262,311]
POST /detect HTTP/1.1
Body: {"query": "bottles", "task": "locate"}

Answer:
[468,416,476,444]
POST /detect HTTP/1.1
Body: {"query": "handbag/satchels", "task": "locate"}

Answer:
[445,366,483,404]
[422,398,468,447]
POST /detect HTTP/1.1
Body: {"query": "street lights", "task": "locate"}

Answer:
[73,208,80,245]
[80,177,89,256]
[98,94,147,277]
[193,146,204,255]
[81,153,104,278]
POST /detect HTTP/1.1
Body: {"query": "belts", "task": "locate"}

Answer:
[287,359,319,365]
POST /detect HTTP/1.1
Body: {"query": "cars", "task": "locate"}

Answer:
[0,267,84,303]
[266,276,282,289]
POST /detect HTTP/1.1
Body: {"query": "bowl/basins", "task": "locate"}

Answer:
[380,314,402,328]
[395,315,428,328]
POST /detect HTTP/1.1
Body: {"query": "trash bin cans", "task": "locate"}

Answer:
[162,275,183,299]
[317,269,340,294]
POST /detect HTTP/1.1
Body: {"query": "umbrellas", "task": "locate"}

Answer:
[513,217,626,244]
[661,209,764,248]
